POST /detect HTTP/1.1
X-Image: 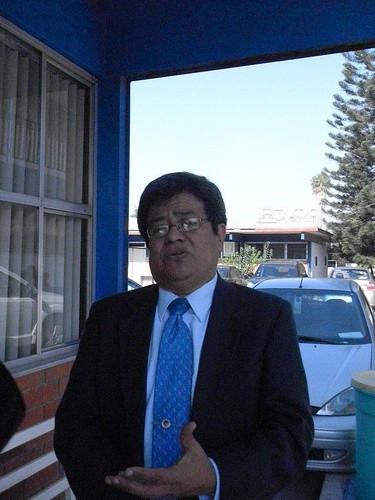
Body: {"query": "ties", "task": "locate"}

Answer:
[150,298,194,500]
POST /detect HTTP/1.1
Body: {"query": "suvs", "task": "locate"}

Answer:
[247,260,307,291]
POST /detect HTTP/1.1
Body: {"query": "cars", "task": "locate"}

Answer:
[216,265,247,287]
[0,267,65,348]
[325,266,375,307]
[248,273,374,473]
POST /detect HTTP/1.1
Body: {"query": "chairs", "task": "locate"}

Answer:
[319,298,352,322]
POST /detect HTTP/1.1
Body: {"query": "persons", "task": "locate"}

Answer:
[53,172,314,500]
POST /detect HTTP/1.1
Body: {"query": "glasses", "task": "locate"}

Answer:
[147,216,210,241]
